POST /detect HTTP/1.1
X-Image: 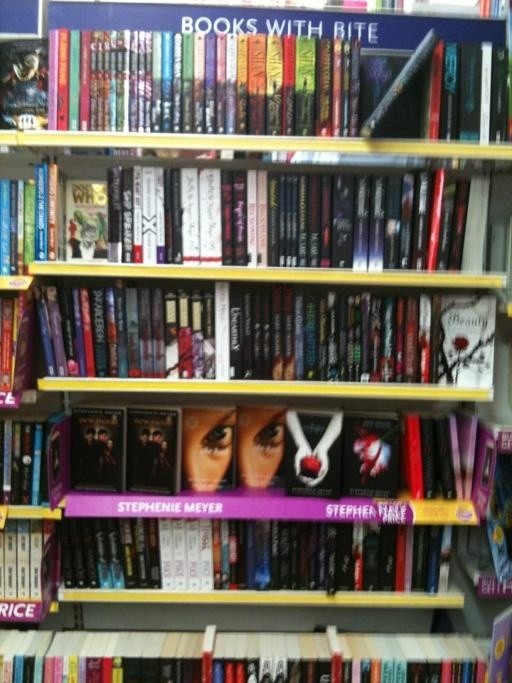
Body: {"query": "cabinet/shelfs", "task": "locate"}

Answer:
[1,0,511,633]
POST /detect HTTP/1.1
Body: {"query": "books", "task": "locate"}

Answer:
[1,149,511,276]
[1,518,454,600]
[1,625,485,683]
[0,26,512,142]
[0,404,512,513]
[1,281,497,393]
